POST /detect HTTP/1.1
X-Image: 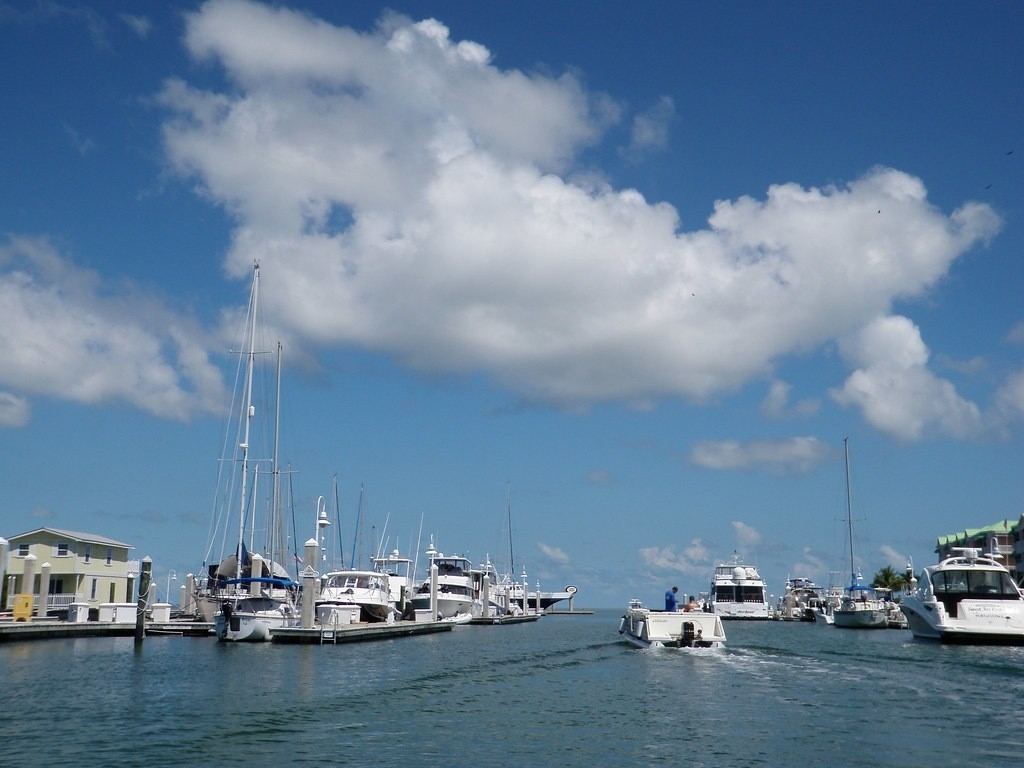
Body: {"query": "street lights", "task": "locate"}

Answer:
[166,569,177,605]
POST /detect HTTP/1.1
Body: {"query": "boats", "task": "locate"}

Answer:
[875,587,910,630]
[811,571,848,624]
[775,577,821,622]
[709,550,774,620]
[620,598,726,647]
[471,567,510,616]
[901,545,1024,646]
[410,552,473,617]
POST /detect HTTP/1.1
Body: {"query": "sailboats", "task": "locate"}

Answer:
[185,255,426,627]
[834,439,891,631]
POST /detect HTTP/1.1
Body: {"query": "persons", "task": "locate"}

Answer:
[664,587,679,611]
[684,595,700,612]
